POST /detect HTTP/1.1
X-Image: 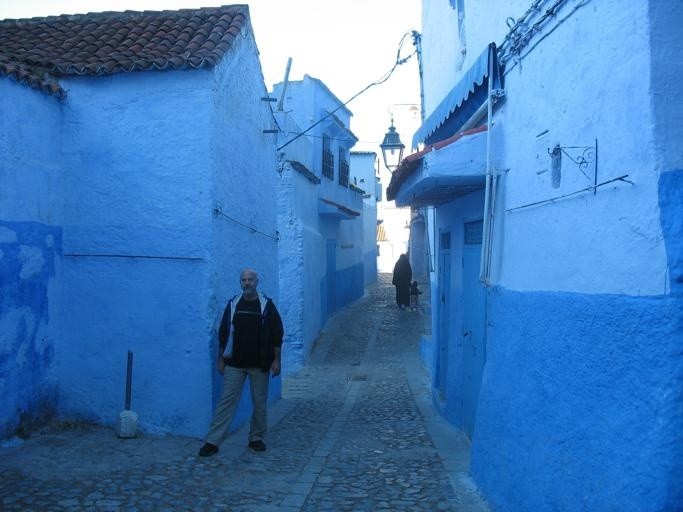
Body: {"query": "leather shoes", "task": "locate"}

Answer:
[249,440,266,451]
[200,443,219,457]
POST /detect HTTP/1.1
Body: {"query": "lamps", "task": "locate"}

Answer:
[548,138,598,195]
[408,104,420,120]
[379,103,422,173]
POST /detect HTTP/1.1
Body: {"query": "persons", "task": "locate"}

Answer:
[409,281,422,305]
[198,269,285,457]
[392,253,412,310]
